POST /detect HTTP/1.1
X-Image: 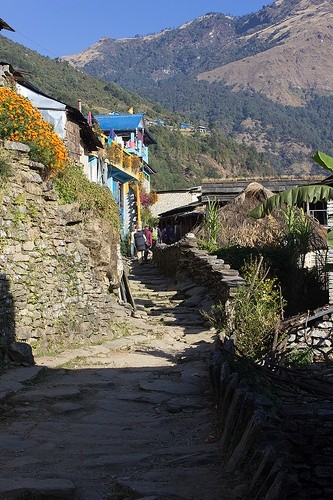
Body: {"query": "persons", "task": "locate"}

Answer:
[134,224,174,265]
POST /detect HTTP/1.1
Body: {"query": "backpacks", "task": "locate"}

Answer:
[134,230,150,251]
